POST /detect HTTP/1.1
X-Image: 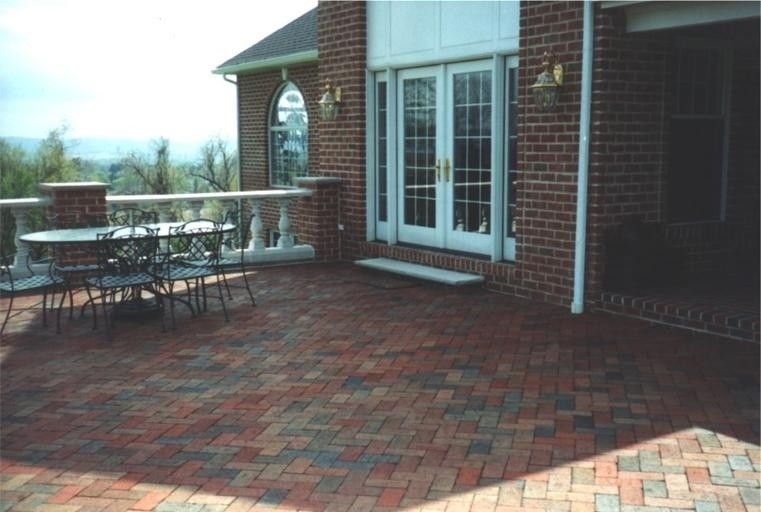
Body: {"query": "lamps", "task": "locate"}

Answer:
[528,52,563,112]
[317,84,342,121]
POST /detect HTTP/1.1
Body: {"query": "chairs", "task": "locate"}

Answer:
[0,207,257,334]
